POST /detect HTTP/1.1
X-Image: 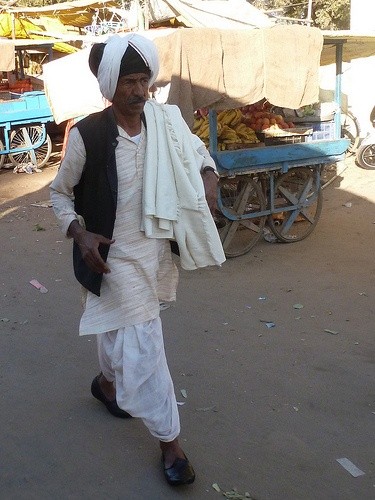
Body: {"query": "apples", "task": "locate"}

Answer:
[197,103,295,131]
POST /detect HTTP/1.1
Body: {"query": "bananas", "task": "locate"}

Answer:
[193,109,260,151]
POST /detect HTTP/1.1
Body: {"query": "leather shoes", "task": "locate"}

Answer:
[91,376,133,418]
[160,449,196,486]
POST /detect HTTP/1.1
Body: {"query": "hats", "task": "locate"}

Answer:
[89,33,160,102]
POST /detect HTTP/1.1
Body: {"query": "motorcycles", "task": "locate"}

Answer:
[354,105,375,169]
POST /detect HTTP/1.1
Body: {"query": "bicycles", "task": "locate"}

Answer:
[323,107,360,159]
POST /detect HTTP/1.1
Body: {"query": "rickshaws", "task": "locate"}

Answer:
[0,39,55,171]
[179,97,351,257]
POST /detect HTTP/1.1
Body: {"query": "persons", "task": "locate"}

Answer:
[47,33,227,488]
